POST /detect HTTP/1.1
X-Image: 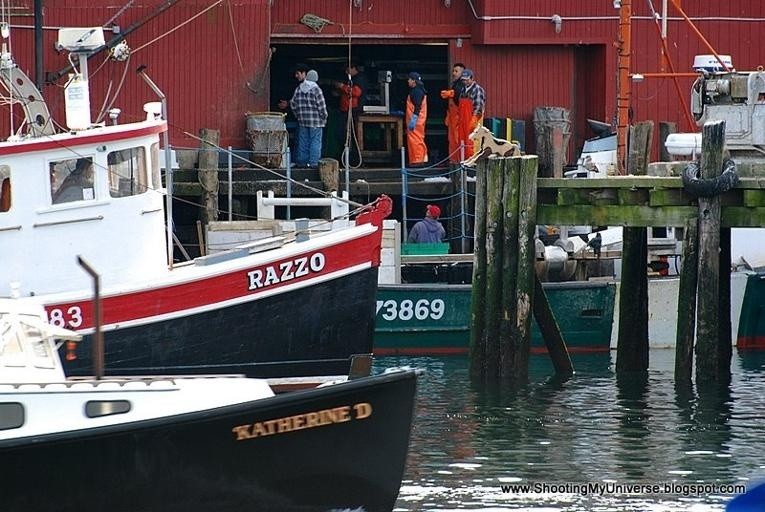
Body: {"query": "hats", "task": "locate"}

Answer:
[426,204,440,217]
[307,70,319,81]
[459,69,472,81]
[409,72,424,85]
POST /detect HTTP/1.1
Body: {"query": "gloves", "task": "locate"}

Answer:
[408,114,417,130]
[440,90,454,98]
[469,115,478,130]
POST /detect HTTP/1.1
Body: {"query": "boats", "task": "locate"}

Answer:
[1,254,424,512]
[204,145,618,359]
[0,0,394,385]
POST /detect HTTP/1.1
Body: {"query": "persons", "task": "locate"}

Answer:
[52,157,94,207]
[290,69,329,169]
[456,69,486,161]
[405,72,429,168]
[332,62,365,146]
[407,204,448,243]
[441,63,468,163]
[278,63,311,168]
